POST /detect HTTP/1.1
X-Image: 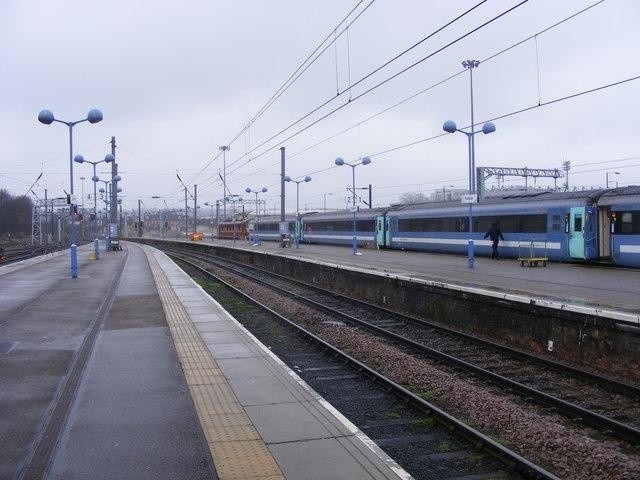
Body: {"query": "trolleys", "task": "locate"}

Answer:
[517,239,551,269]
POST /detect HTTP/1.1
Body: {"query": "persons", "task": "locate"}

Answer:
[483,221,505,261]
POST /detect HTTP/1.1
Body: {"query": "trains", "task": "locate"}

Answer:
[217,187,640,272]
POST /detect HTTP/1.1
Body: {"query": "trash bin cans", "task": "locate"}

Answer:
[280,233,291,248]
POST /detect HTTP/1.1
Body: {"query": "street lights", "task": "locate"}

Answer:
[562,160,571,191]
[179,196,244,243]
[218,144,231,219]
[440,120,492,268]
[334,157,373,256]
[246,186,267,247]
[460,58,479,199]
[605,172,621,189]
[91,175,124,251]
[71,153,114,261]
[38,106,103,279]
[283,176,311,249]
